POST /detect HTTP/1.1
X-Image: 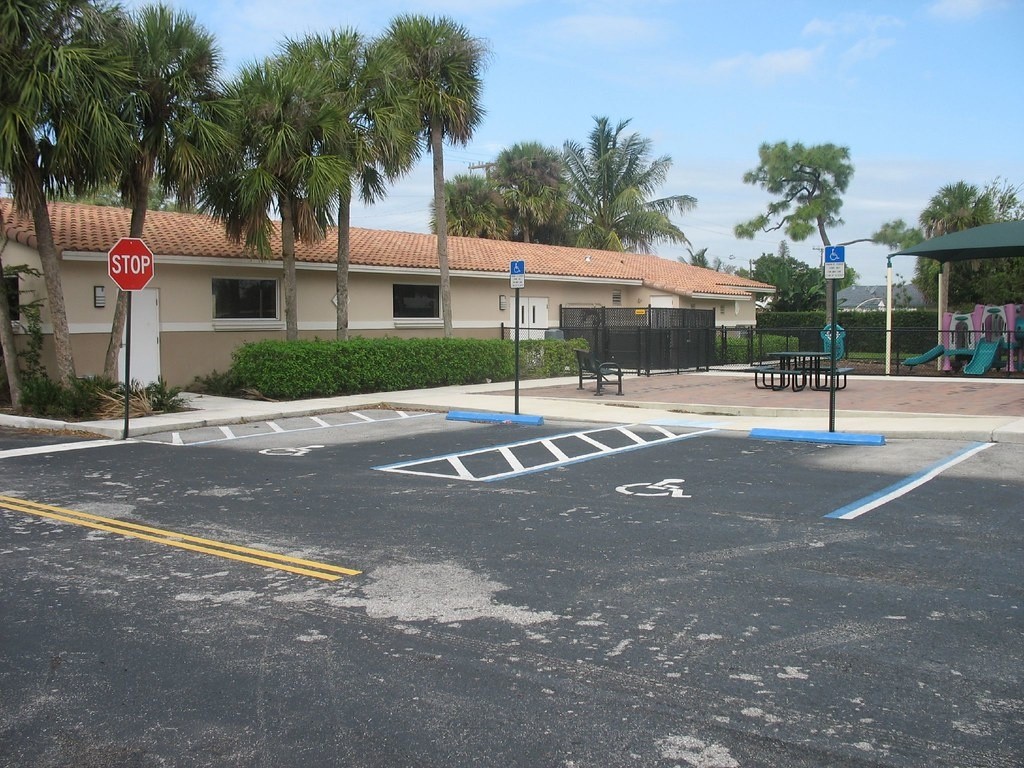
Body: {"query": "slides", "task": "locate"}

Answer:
[900,343,944,366]
[963,336,1005,377]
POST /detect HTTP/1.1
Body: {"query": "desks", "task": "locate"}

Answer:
[767,351,831,386]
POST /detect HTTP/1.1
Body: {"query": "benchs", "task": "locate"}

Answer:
[794,366,831,387]
[746,365,775,388]
[574,349,623,397]
[824,367,855,392]
[761,368,805,392]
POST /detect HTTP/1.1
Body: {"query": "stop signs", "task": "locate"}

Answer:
[108,238,154,291]
